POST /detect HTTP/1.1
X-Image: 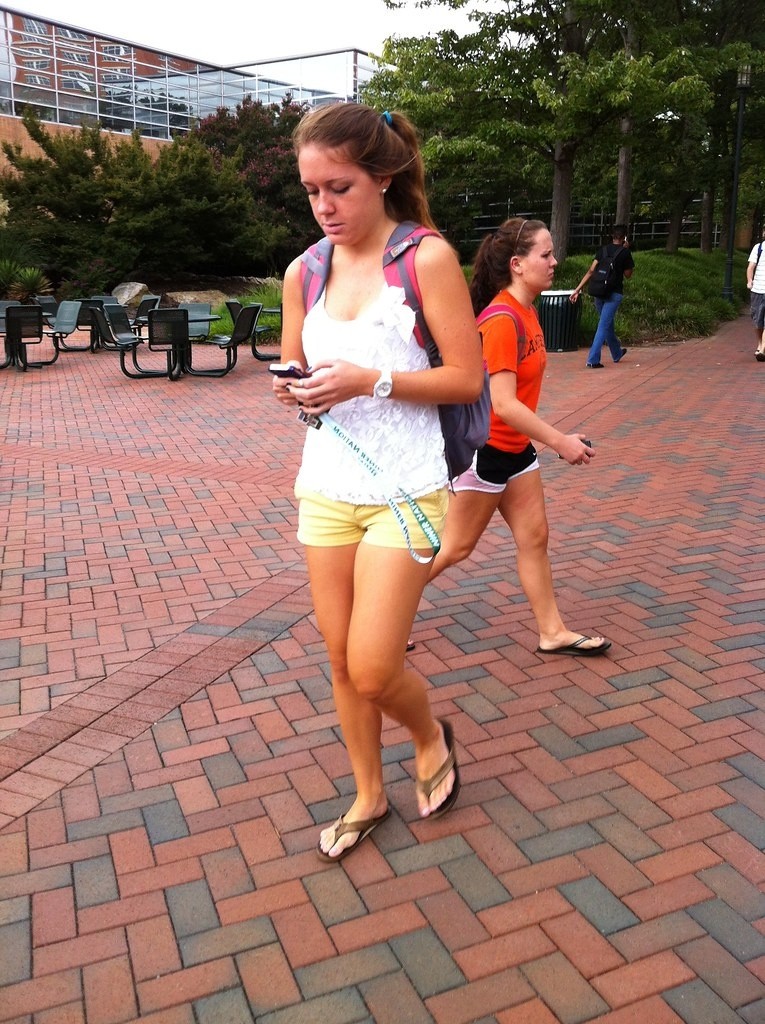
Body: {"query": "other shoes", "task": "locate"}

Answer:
[586,363,604,368]
[614,349,627,362]
[754,350,765,362]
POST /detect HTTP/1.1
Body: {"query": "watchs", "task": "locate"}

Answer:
[370,369,392,402]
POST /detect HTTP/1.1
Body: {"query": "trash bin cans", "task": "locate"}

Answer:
[538,289,582,353]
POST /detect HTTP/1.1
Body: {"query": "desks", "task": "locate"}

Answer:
[261,308,281,313]
[0,312,52,319]
[137,315,222,322]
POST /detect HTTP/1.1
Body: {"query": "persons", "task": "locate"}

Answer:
[271,103,482,863]
[406,216,610,655]
[568,224,635,368]
[747,225,765,362]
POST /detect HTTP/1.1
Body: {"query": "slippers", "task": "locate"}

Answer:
[536,633,612,657]
[316,804,391,863]
[416,718,460,820]
[405,644,416,652]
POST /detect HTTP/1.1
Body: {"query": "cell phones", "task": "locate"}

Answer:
[557,439,591,459]
[268,363,308,379]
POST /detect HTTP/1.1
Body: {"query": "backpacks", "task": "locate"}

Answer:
[588,245,624,300]
[298,218,491,482]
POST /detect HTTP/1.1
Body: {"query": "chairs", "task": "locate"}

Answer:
[0,296,281,381]
